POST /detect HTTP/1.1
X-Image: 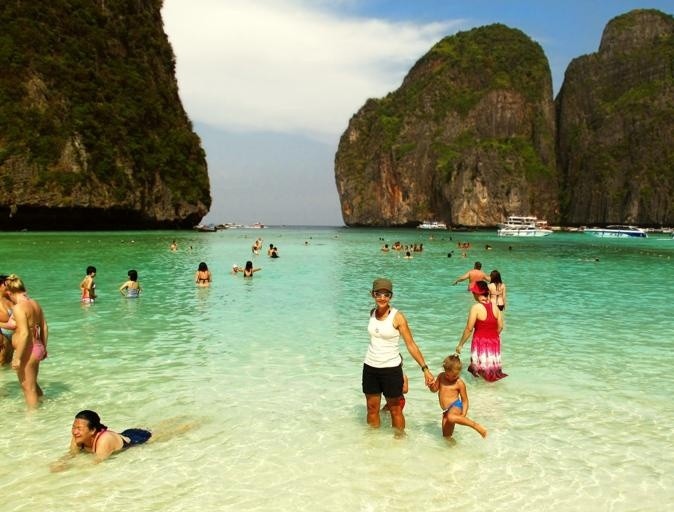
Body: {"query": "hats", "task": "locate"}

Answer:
[373,277,392,292]
[468,282,490,294]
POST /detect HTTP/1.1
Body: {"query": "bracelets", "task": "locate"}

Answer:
[422,365,428,371]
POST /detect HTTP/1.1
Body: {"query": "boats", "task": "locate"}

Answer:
[192,223,243,232]
[415,220,446,231]
[584,225,648,239]
[244,222,269,228]
[496,215,553,236]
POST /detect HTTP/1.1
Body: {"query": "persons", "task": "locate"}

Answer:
[253,238,279,258]
[47,408,205,472]
[196,262,211,285]
[381,352,409,417]
[427,355,489,440]
[119,270,142,297]
[0,275,16,366]
[455,280,508,382]
[361,278,434,441]
[80,266,96,303]
[454,262,490,291]
[383,235,491,258]
[237,261,261,277]
[171,241,176,250]
[487,270,505,312]
[4,272,49,411]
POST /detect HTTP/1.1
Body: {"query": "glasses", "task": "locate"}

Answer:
[373,290,392,299]
